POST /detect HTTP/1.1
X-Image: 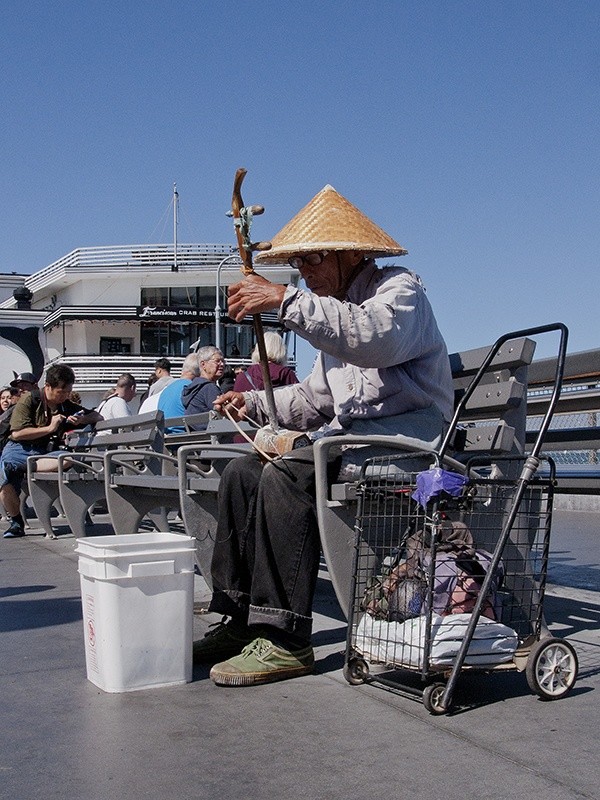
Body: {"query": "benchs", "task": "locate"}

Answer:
[20,337,600,640]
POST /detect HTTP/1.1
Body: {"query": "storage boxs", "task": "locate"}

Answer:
[68,531,198,692]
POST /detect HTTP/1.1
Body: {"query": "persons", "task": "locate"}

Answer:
[0,332,299,539]
[188,185,459,686]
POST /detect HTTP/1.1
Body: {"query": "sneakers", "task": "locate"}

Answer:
[189,614,251,666]
[4,461,23,474]
[3,521,26,537]
[209,636,315,686]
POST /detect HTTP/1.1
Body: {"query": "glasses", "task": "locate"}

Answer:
[288,249,333,269]
[207,359,226,365]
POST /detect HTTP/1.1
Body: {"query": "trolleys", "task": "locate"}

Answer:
[342,322,578,715]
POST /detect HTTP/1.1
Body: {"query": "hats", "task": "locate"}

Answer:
[253,184,408,264]
[10,369,38,387]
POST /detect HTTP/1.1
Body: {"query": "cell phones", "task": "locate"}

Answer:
[65,411,83,423]
